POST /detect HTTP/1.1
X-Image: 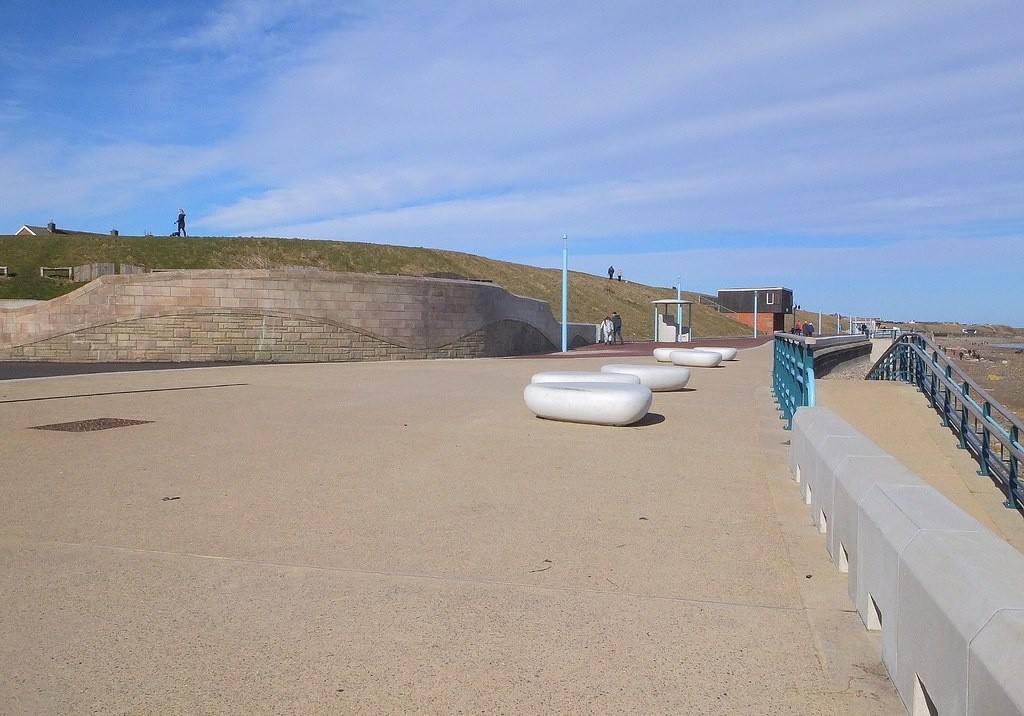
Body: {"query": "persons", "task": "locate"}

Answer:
[808,322,814,336]
[953,349,956,355]
[175,209,187,237]
[802,321,810,335]
[611,311,624,345]
[862,323,867,330]
[617,269,622,281]
[794,320,803,335]
[600,315,614,345]
[959,352,963,359]
[968,350,971,355]
[608,266,614,280]
